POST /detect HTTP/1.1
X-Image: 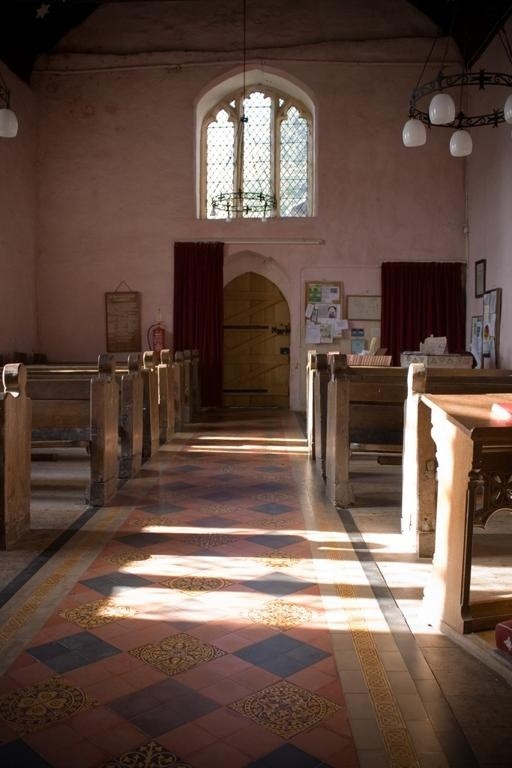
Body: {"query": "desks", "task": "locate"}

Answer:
[419,390,512,685]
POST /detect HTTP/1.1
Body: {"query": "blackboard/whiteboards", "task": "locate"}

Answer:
[105,291,142,353]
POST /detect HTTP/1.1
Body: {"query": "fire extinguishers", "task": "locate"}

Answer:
[147,321,165,360]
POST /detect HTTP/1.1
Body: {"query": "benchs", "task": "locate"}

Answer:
[310,352,510,508]
[1,349,193,549]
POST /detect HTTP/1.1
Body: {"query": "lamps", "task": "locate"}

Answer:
[401,0,511,159]
[0,81,19,139]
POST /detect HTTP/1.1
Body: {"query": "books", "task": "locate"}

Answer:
[491,402,511,419]
[348,354,392,366]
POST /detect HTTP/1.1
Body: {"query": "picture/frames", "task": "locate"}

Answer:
[474,259,485,298]
